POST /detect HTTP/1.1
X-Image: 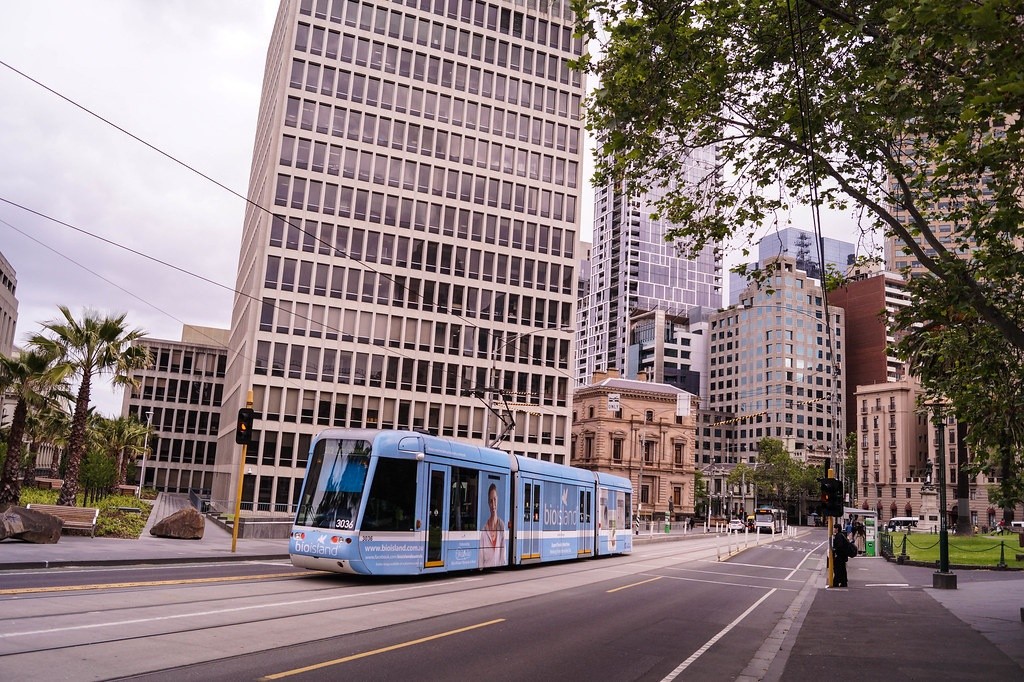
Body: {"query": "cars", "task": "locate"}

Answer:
[728,520,745,533]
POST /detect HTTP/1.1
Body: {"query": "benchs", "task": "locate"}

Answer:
[27,503,100,539]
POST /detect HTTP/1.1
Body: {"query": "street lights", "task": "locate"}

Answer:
[924,403,955,573]
[138,412,154,499]
[727,438,748,463]
[735,304,758,310]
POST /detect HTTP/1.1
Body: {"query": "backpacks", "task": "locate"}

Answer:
[841,532,858,558]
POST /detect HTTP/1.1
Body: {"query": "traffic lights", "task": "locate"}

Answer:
[820,478,836,515]
[236,409,253,445]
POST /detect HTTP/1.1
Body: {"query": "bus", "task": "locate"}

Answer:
[753,509,787,533]
[288,429,632,577]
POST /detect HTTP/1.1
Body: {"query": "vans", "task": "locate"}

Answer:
[888,517,919,532]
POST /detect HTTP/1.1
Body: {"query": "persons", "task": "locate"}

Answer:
[892,524,896,532]
[845,522,863,557]
[827,524,848,588]
[748,520,754,533]
[684,516,694,530]
[884,522,888,531]
[996,518,1005,535]
[480,483,506,568]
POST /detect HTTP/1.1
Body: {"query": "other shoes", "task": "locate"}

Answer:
[833,584,839,587]
[840,584,847,587]
